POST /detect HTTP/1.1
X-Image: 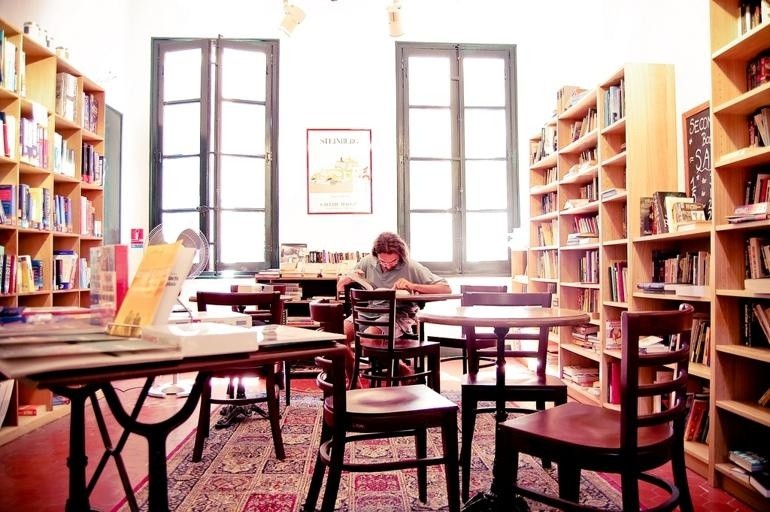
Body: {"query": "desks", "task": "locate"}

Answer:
[190,294,291,429]
[0,324,354,512]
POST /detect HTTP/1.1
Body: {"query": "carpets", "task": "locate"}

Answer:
[110,392,652,512]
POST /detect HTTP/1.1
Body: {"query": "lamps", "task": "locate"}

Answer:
[279,0,306,37]
[384,0,405,37]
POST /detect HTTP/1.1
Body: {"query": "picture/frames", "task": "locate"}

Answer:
[305,127,373,214]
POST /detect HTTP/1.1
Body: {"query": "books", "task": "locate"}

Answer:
[108,240,195,337]
[18,404,38,416]
[89,243,130,326]
[349,272,410,296]
[53,395,70,405]
[726,2,770,500]
[520,78,626,409]
[256,242,369,328]
[0,309,260,380]
[0,19,106,325]
[637,189,712,447]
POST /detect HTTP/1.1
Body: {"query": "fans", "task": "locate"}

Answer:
[144,220,211,398]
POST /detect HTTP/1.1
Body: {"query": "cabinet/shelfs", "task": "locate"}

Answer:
[509,248,528,294]
[0,14,106,446]
[709,0,770,512]
[528,62,675,416]
[254,276,418,378]
[632,225,711,479]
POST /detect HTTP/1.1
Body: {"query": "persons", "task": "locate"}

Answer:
[337,230,452,389]
